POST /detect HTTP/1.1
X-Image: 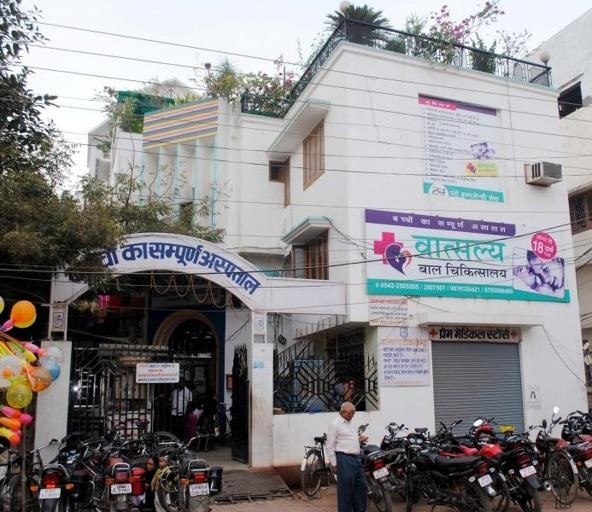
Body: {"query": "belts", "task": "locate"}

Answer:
[337,451,358,457]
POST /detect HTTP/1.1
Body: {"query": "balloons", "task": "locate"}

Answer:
[0,295,64,446]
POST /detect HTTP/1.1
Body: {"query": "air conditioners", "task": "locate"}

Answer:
[526,160,563,187]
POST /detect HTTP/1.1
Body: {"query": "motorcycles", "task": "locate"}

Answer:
[0,419,225,512]
[299,403,592,511]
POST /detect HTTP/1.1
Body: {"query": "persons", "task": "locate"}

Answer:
[324,401,369,512]
[515,261,557,296]
[183,401,204,439]
[150,457,171,511]
[169,378,193,435]
[144,456,158,509]
[525,251,563,290]
[199,392,219,448]
